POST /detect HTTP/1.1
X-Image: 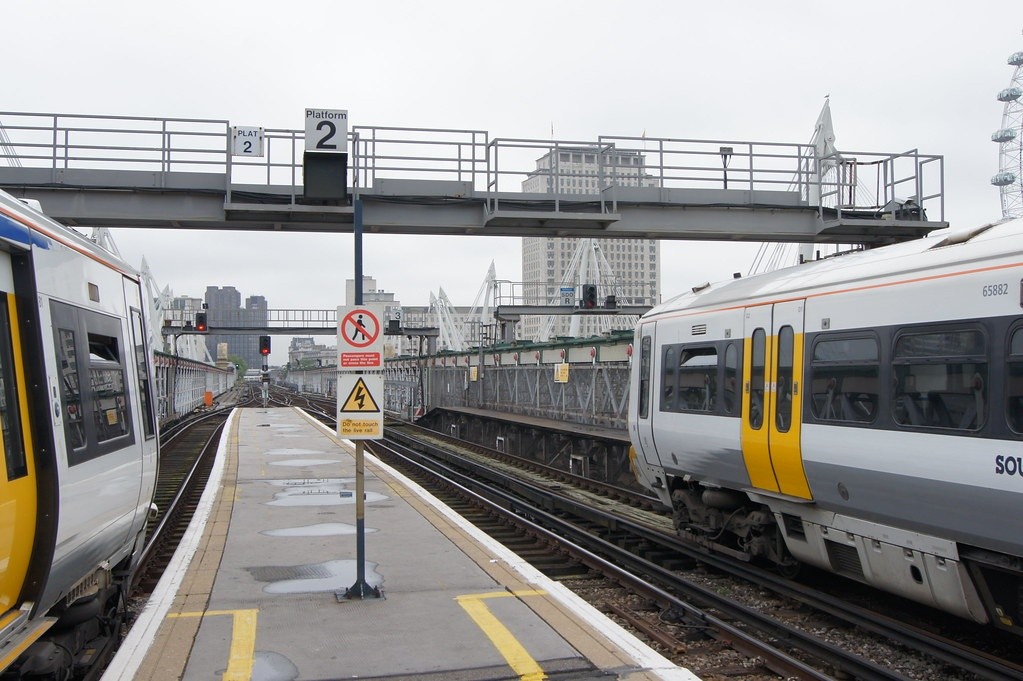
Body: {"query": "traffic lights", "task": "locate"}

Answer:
[196,313,207,331]
[259,336,270,355]
[583,284,597,308]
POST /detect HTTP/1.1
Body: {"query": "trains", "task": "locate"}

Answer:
[628,215,1023,637]
[0,187,160,681]
